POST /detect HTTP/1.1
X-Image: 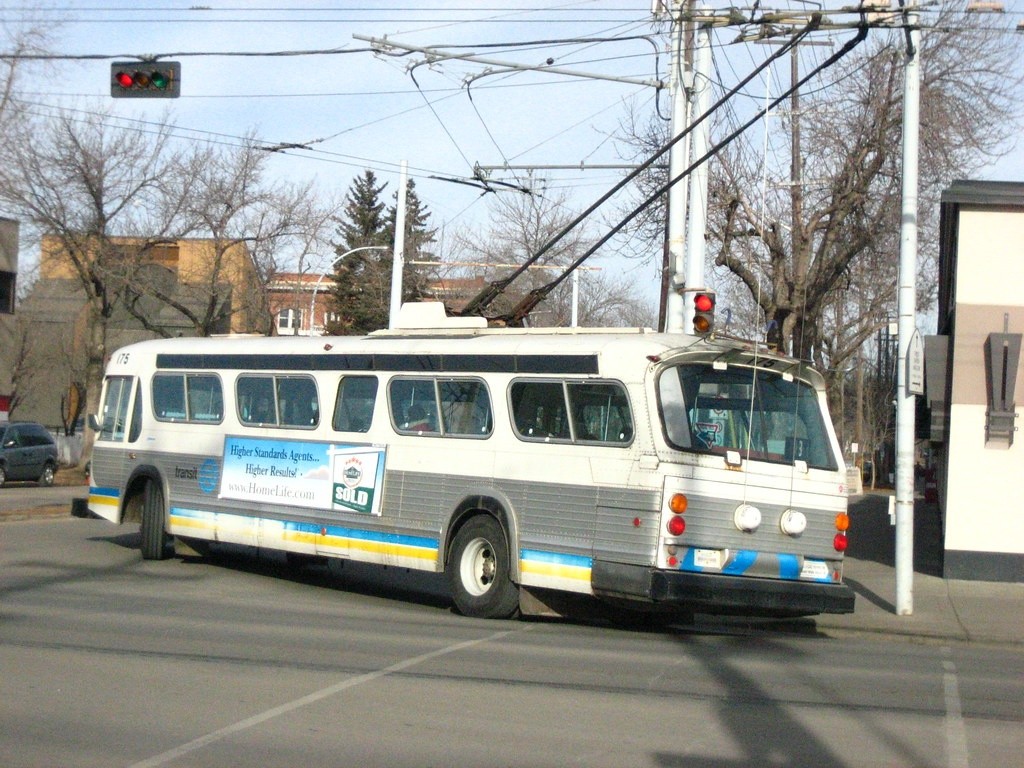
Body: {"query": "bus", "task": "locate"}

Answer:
[71,301,856,620]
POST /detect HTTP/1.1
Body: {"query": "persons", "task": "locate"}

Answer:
[400,405,434,431]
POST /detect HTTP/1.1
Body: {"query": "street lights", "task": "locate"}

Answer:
[309,246,389,336]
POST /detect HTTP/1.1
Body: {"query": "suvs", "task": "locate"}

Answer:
[0,419,58,487]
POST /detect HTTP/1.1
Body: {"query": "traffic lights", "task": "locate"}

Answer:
[693,293,715,336]
[111,62,181,98]
